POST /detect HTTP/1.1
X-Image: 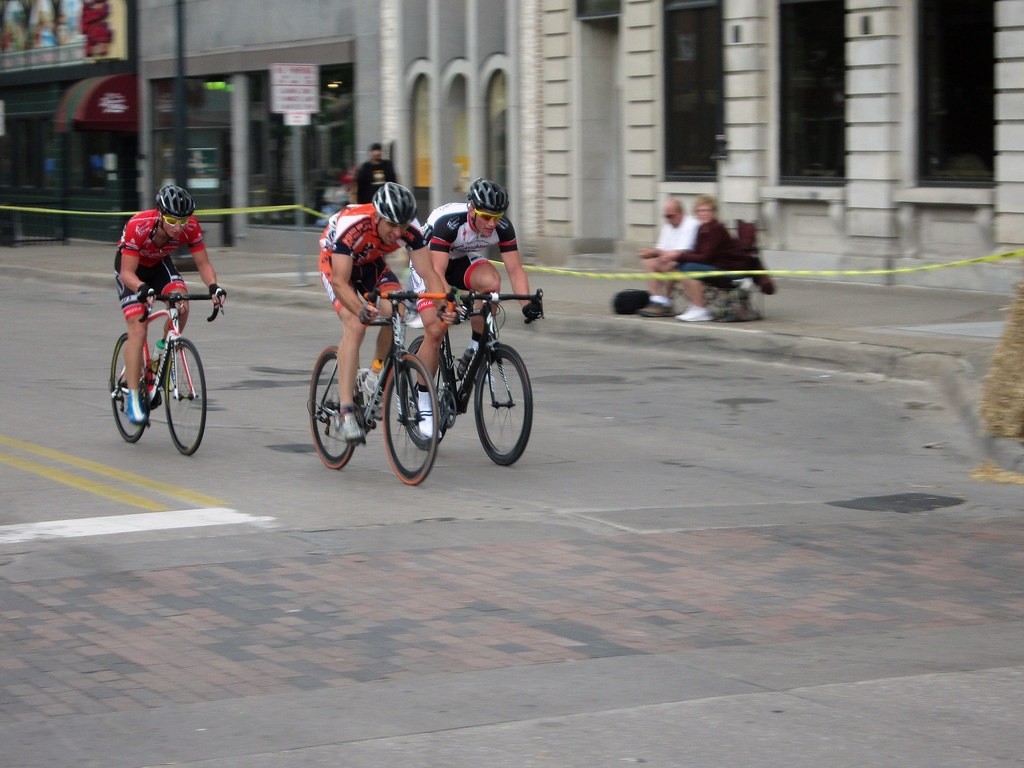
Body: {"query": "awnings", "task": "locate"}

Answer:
[53,70,138,136]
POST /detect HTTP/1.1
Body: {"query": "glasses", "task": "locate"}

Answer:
[474,208,505,222]
[159,213,189,226]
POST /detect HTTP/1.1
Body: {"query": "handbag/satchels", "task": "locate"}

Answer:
[615,290,651,316]
[705,286,763,323]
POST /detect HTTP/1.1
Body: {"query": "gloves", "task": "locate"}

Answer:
[522,303,541,320]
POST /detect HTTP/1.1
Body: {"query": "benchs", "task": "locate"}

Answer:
[668,246,763,323]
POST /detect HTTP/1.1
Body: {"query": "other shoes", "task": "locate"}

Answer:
[419,421,442,439]
[151,355,175,390]
[126,388,146,422]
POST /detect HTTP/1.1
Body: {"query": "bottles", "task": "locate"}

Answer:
[457,348,474,381]
[365,358,384,395]
[150,338,166,375]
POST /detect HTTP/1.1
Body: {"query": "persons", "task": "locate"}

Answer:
[356,143,398,204]
[319,181,467,443]
[409,176,543,439]
[113,184,228,425]
[658,193,733,321]
[635,197,703,317]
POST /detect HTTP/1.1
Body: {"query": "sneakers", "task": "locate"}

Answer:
[675,305,715,321]
[335,407,363,439]
[639,302,674,316]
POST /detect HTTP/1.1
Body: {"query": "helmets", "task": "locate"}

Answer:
[156,184,194,218]
[372,181,417,227]
[467,177,509,214]
